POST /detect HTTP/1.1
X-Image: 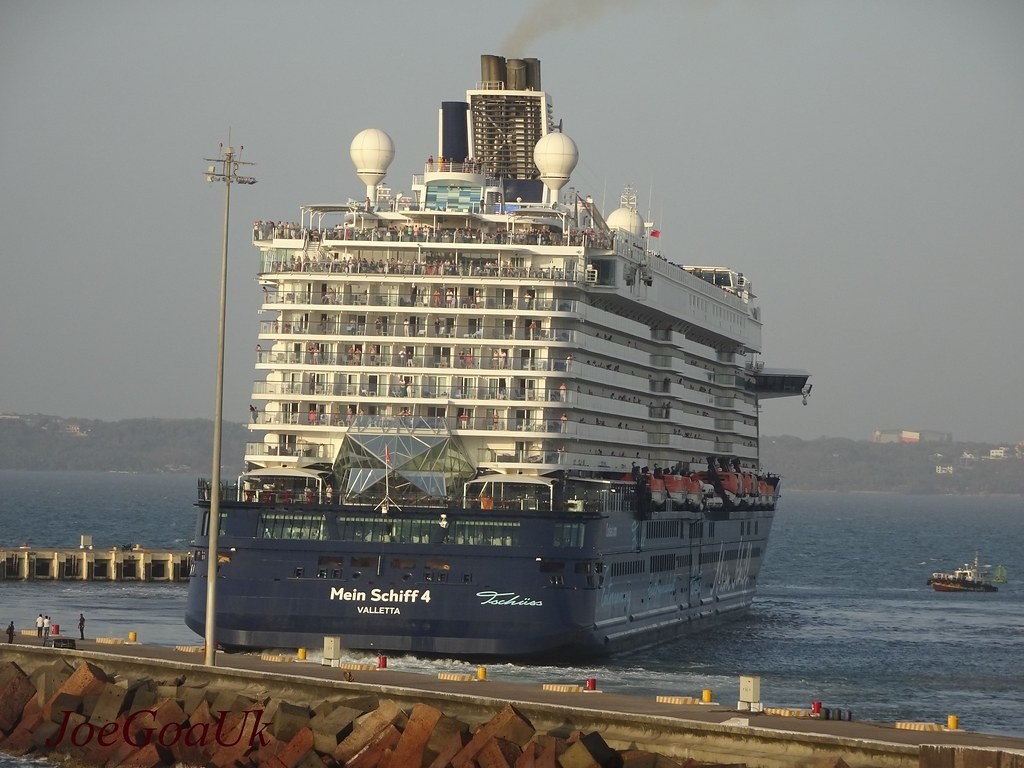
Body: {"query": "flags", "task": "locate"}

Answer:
[386,446,392,465]
[650,229,660,238]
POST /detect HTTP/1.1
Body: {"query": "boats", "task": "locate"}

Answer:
[753,367,811,393]
[928,548,999,594]
[622,474,669,507]
[687,478,708,511]
[703,482,724,510]
[656,475,689,504]
[734,471,776,509]
[693,471,738,505]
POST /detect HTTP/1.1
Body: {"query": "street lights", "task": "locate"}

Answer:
[195,140,257,670]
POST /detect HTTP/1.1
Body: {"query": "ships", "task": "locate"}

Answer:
[183,53,813,669]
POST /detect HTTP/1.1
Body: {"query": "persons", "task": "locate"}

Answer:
[365,196,395,212]
[77,613,86,640]
[479,197,485,214]
[6,621,15,643]
[43,615,51,639]
[249,218,585,434]
[428,155,434,171]
[586,227,617,250]
[556,333,757,472]
[35,614,44,638]
[304,484,333,504]
